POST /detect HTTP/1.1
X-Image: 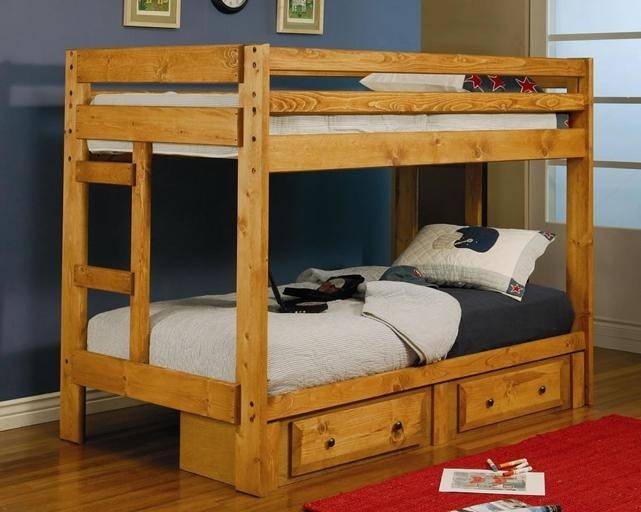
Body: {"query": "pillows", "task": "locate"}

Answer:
[379,220,558,303]
[359,64,546,93]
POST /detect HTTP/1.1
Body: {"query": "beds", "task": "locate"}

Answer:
[59,41,595,496]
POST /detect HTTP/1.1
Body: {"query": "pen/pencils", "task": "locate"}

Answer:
[487,458,533,476]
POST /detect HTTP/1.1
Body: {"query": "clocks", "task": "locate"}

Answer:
[211,1,250,16]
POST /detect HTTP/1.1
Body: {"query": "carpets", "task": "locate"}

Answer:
[303,411,641,511]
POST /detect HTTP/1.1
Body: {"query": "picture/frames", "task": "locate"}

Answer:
[122,1,182,31]
[276,1,325,35]
